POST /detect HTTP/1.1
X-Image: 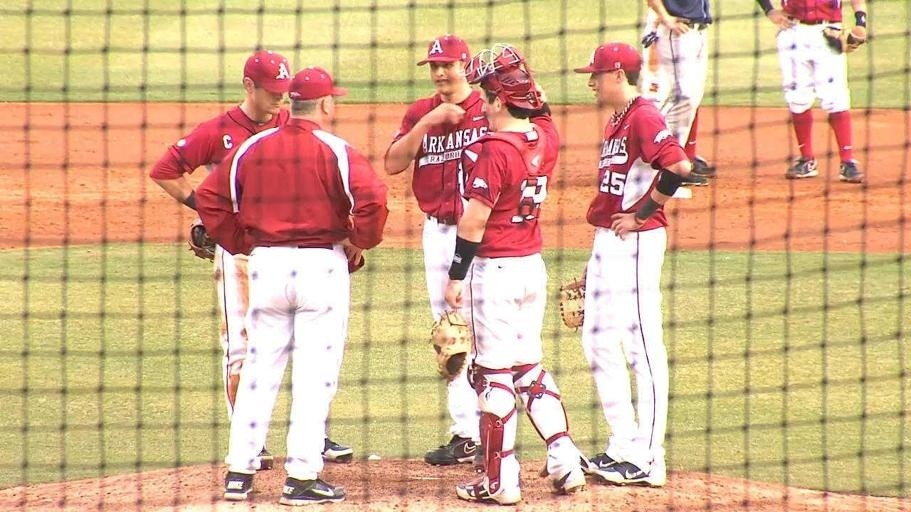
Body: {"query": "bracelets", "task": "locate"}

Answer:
[183,189,199,212]
[755,0,775,14]
[448,234,481,280]
[853,10,867,28]
[635,198,663,222]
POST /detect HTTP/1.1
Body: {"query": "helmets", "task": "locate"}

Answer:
[463,43,544,111]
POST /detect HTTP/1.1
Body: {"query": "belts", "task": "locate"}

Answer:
[685,22,708,30]
[426,214,457,225]
[787,16,836,25]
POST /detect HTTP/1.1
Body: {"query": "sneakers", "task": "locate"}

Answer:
[670,155,865,186]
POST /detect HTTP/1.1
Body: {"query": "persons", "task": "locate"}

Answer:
[753,0,869,186]
[442,45,587,507]
[573,40,694,492]
[192,65,389,506]
[149,48,359,469]
[382,33,547,469]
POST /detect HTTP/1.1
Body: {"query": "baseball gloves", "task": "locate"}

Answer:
[559,282,585,330]
[189,218,216,260]
[825,26,867,53]
[431,311,471,381]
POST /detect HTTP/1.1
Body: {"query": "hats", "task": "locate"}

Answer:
[288,66,346,99]
[244,50,293,94]
[573,42,642,73]
[417,35,470,66]
